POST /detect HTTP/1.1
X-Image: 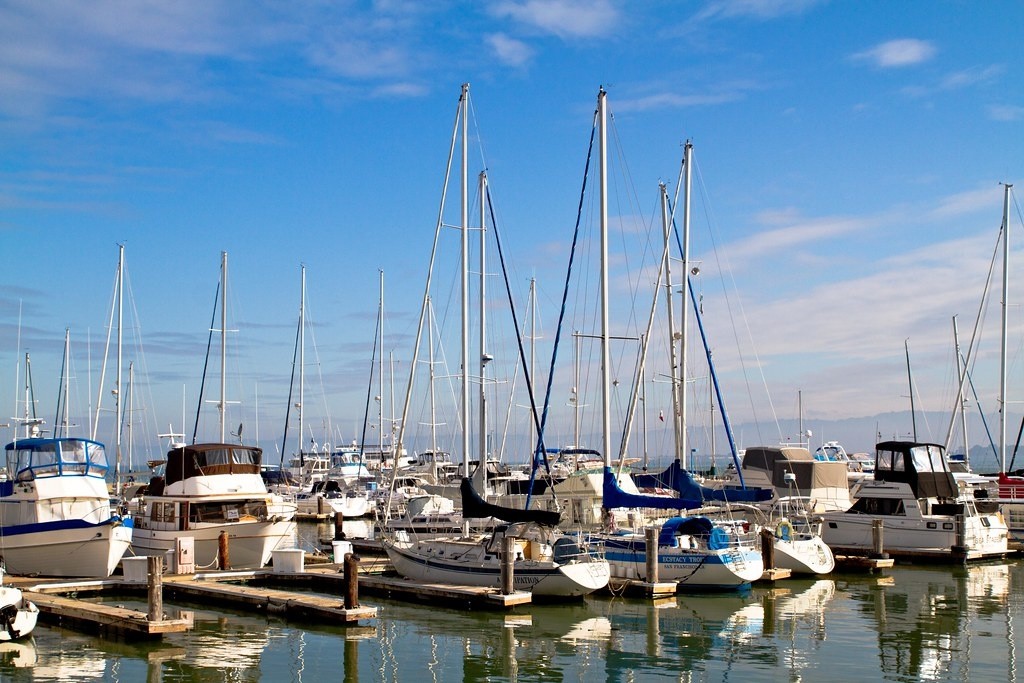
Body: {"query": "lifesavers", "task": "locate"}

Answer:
[775,521,793,538]
[606,511,615,529]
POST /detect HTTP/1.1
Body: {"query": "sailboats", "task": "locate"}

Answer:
[302,83,836,594]
[743,181,1022,558]
[268,266,375,516]
[125,251,297,565]
[0,298,133,576]
